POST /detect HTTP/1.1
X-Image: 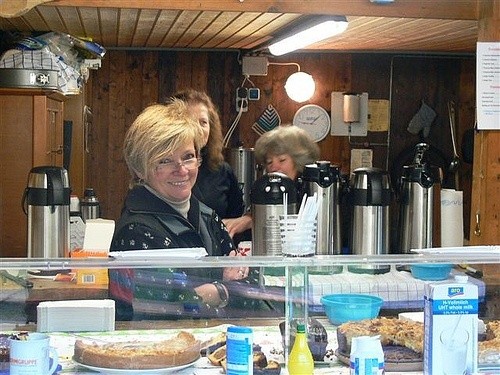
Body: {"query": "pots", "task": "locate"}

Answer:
[0,67,60,90]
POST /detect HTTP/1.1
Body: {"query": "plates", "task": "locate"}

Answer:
[70,354,201,375]
[0,356,62,375]
[335,345,423,372]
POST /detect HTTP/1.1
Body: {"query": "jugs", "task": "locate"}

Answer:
[22,165,73,276]
[80,188,100,221]
[396,162,433,272]
[250,172,300,276]
[296,161,343,275]
[342,166,394,274]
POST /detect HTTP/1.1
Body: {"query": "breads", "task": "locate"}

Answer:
[200,332,282,371]
[337,318,424,357]
[478,320,500,362]
[74,331,201,370]
[279,318,328,361]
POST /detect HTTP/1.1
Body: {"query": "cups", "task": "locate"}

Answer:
[9,332,59,375]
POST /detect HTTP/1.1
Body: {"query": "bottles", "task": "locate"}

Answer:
[287,325,314,375]
[70,194,85,251]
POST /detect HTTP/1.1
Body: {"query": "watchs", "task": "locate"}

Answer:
[213,281,229,312]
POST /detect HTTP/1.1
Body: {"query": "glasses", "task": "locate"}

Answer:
[155,156,201,172]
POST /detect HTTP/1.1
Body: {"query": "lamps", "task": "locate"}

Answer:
[267,15,349,57]
[269,62,315,103]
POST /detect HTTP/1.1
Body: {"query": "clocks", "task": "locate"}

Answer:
[292,104,330,143]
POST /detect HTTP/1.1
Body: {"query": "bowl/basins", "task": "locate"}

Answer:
[320,294,383,327]
[410,264,455,281]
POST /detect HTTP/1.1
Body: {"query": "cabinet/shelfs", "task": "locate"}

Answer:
[0,84,90,258]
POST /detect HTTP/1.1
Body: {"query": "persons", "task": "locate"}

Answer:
[107,98,266,322]
[217,125,328,255]
[150,87,247,251]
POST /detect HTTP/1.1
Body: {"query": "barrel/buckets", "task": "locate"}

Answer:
[220,147,259,215]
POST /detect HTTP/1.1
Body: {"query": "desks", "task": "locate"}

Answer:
[240,265,486,312]
